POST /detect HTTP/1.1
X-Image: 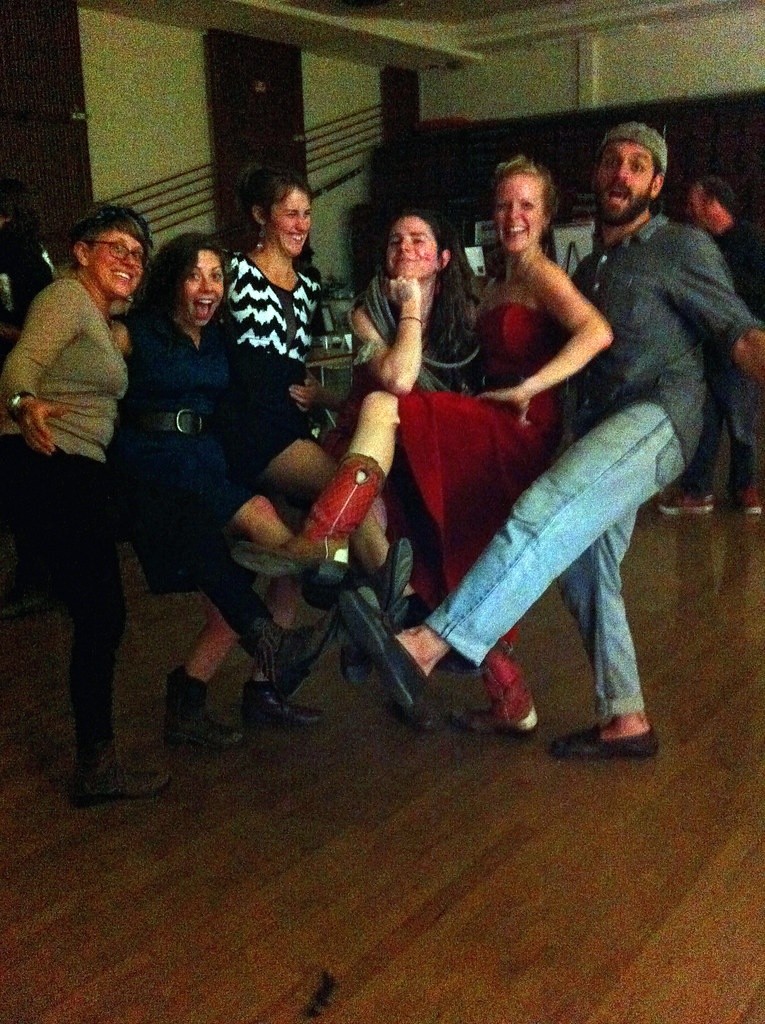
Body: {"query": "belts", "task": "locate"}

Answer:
[118,407,224,434]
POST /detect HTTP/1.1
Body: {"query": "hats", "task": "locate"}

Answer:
[595,121,668,176]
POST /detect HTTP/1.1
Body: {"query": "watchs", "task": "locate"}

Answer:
[7,392,35,422]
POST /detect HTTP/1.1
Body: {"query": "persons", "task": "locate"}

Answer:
[164,157,413,738]
[104,230,340,699]
[658,177,761,514]
[234,155,614,732]
[0,203,154,805]
[347,206,494,625]
[335,120,765,762]
[0,201,59,337]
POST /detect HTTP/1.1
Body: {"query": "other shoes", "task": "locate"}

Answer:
[656,490,717,516]
[1,583,61,618]
[65,756,171,809]
[721,486,761,514]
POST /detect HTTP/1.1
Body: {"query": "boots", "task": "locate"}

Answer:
[230,452,387,585]
[445,627,539,737]
[238,602,338,696]
[394,592,488,681]
[161,665,244,752]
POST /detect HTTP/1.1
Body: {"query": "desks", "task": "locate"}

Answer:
[307,346,355,430]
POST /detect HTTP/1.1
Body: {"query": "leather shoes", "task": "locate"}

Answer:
[549,722,661,758]
[354,537,414,626]
[339,590,426,708]
[341,631,372,686]
[239,690,328,729]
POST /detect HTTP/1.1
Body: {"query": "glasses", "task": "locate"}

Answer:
[80,240,149,266]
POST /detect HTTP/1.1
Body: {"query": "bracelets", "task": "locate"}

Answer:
[397,316,425,327]
